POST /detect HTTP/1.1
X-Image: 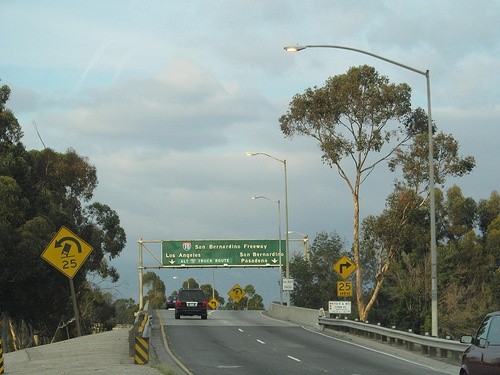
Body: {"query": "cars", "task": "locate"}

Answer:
[459,310,500,375]
[164,295,177,310]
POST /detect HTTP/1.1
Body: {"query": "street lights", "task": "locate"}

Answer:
[172,276,189,289]
[284,231,309,261]
[283,42,439,336]
[250,195,283,305]
[244,151,290,306]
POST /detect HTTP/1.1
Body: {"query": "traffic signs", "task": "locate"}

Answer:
[161,240,286,267]
[40,226,93,280]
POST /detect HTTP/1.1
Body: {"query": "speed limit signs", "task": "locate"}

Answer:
[337,281,352,297]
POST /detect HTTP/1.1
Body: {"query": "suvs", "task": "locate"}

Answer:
[174,288,209,320]
